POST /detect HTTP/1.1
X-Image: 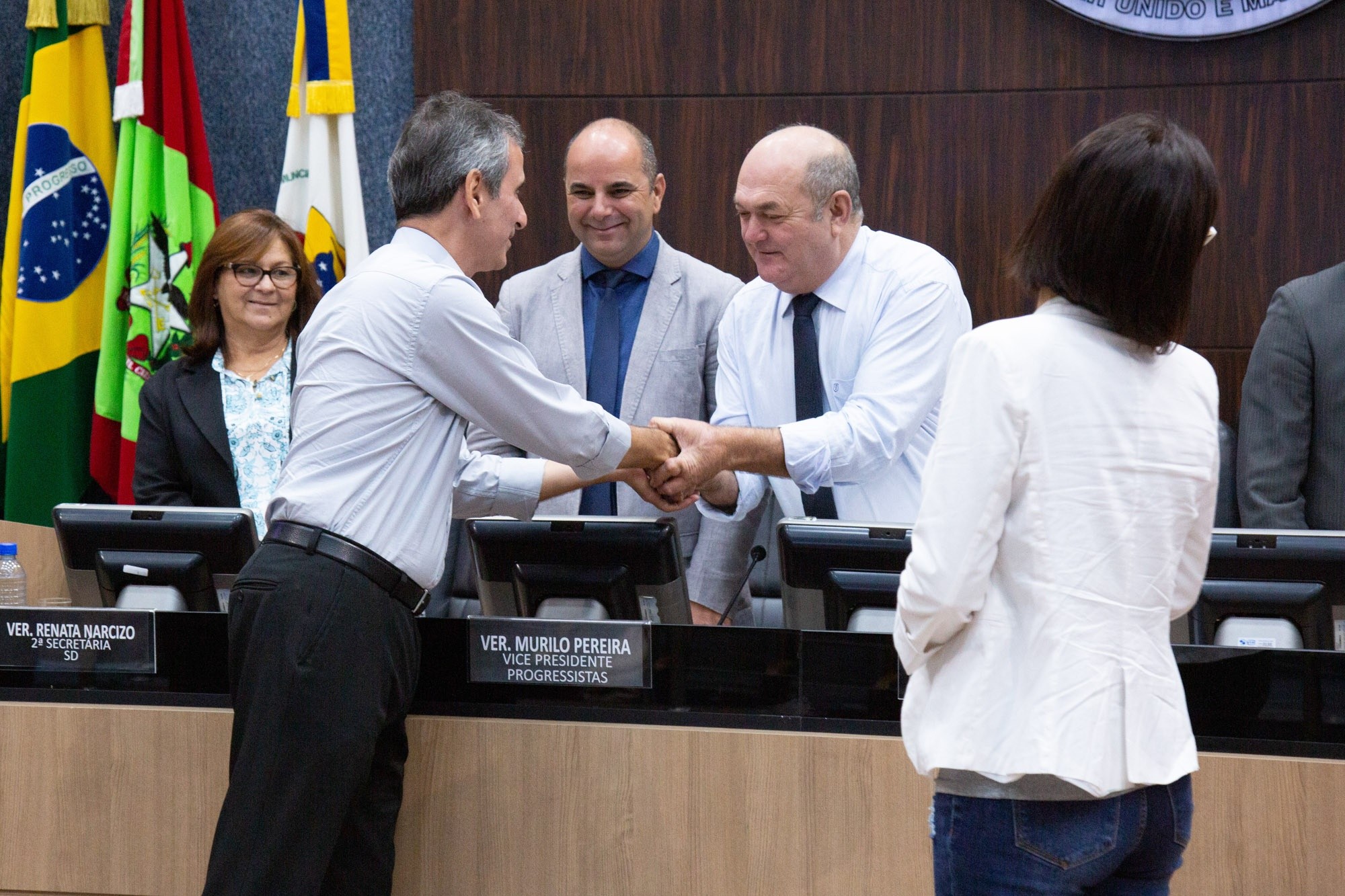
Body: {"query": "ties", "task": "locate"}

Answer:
[578,269,635,515]
[791,291,840,520]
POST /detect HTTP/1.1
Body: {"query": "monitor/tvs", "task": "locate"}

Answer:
[776,517,914,634]
[464,513,694,623]
[1169,528,1345,651]
[52,503,258,613]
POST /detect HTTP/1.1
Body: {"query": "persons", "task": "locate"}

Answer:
[200,91,695,895]
[1236,263,1345,533]
[462,116,771,627]
[132,209,322,546]
[891,116,1220,896]
[652,124,973,634]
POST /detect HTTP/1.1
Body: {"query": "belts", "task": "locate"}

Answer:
[260,521,432,617]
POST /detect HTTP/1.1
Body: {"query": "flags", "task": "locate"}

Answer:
[0,0,118,528]
[90,1,220,505]
[273,0,370,302]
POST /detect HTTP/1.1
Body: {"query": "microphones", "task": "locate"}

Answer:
[717,545,767,625]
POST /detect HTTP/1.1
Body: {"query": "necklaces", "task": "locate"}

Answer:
[225,336,288,377]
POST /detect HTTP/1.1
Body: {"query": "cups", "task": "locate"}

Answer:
[36,597,72,607]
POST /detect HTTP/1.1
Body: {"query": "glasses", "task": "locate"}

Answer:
[222,262,301,289]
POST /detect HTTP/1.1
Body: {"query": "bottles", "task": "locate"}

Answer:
[0,543,27,608]
